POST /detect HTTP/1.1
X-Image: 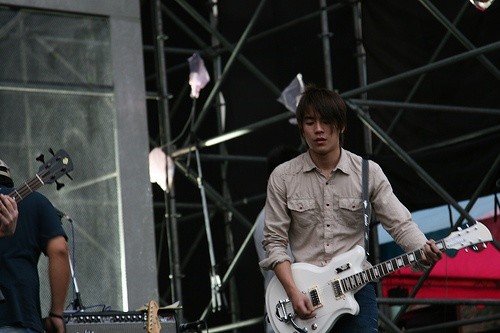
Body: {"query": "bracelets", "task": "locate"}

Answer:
[49,312,64,321]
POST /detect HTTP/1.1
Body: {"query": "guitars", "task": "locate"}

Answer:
[0,147,75,215]
[264,220,493,333]
[143,300,162,333]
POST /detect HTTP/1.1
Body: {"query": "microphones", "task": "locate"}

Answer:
[56,210,73,222]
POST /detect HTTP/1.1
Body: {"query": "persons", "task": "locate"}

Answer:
[0,156,72,332]
[259,85,444,332]
